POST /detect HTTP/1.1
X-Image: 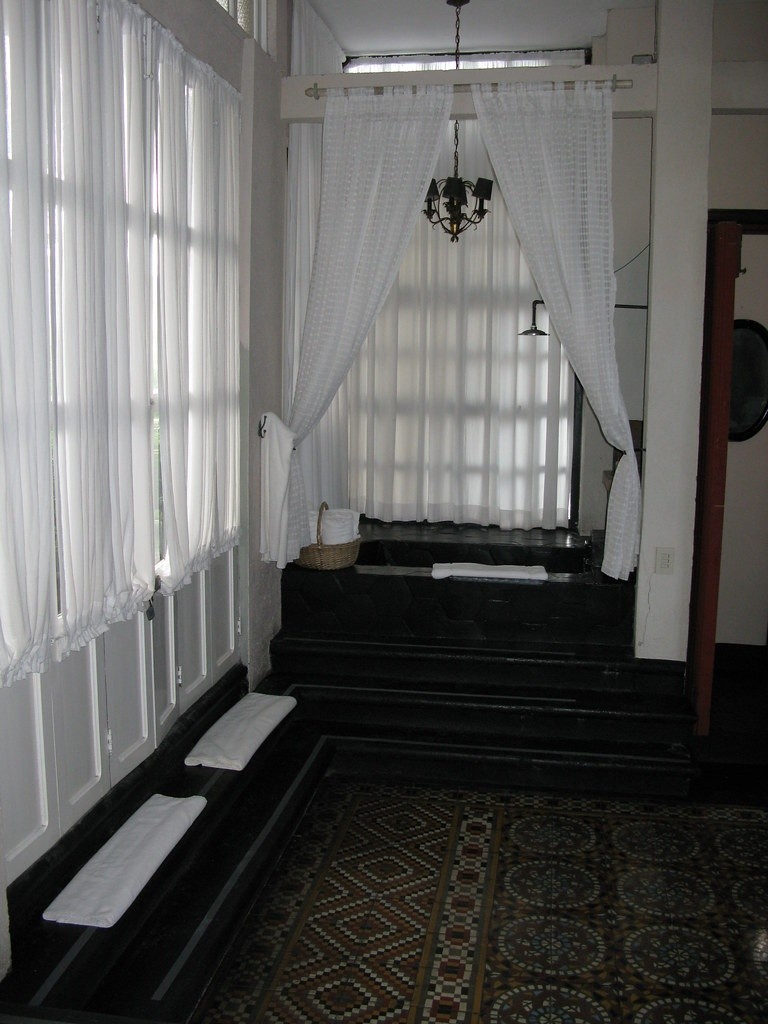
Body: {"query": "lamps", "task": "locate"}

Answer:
[423,0,494,243]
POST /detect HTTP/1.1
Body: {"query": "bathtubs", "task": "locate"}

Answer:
[280,522,640,628]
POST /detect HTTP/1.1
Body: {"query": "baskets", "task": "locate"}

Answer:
[294,502,363,570]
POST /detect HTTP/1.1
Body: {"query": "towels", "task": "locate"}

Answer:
[260,412,296,569]
[185,692,298,772]
[432,562,549,581]
[308,508,361,545]
[42,793,208,929]
